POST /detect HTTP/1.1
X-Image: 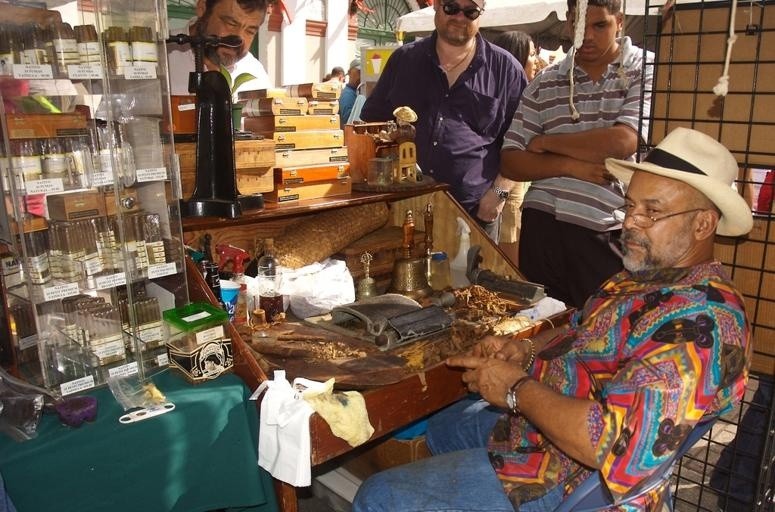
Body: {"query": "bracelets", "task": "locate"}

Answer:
[520,335,536,371]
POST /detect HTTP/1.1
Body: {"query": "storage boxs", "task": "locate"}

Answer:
[165,301,230,382]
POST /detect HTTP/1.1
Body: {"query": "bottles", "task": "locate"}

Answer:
[257,238,284,324]
[430,252,451,291]
[205,263,227,311]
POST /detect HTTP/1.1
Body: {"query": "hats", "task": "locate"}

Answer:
[605,128,753,235]
[350,59,360,70]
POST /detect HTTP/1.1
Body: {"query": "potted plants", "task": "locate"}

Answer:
[220,63,257,132]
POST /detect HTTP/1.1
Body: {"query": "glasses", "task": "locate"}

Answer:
[439,0,482,20]
[610,205,707,228]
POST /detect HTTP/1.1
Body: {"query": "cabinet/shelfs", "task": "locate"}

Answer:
[1,1,190,400]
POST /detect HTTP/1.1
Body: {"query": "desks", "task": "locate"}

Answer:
[171,181,576,512]
[0,368,277,511]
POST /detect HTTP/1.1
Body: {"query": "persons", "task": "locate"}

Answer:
[360,0,529,240]
[352,127,754,512]
[339,59,361,124]
[323,66,345,89]
[166,0,273,95]
[498,0,655,309]
[496,30,540,267]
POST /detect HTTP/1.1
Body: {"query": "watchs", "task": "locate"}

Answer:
[491,184,510,201]
[505,375,535,414]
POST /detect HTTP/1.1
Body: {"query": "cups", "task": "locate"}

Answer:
[220,280,241,329]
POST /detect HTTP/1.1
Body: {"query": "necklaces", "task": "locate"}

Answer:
[435,35,476,72]
